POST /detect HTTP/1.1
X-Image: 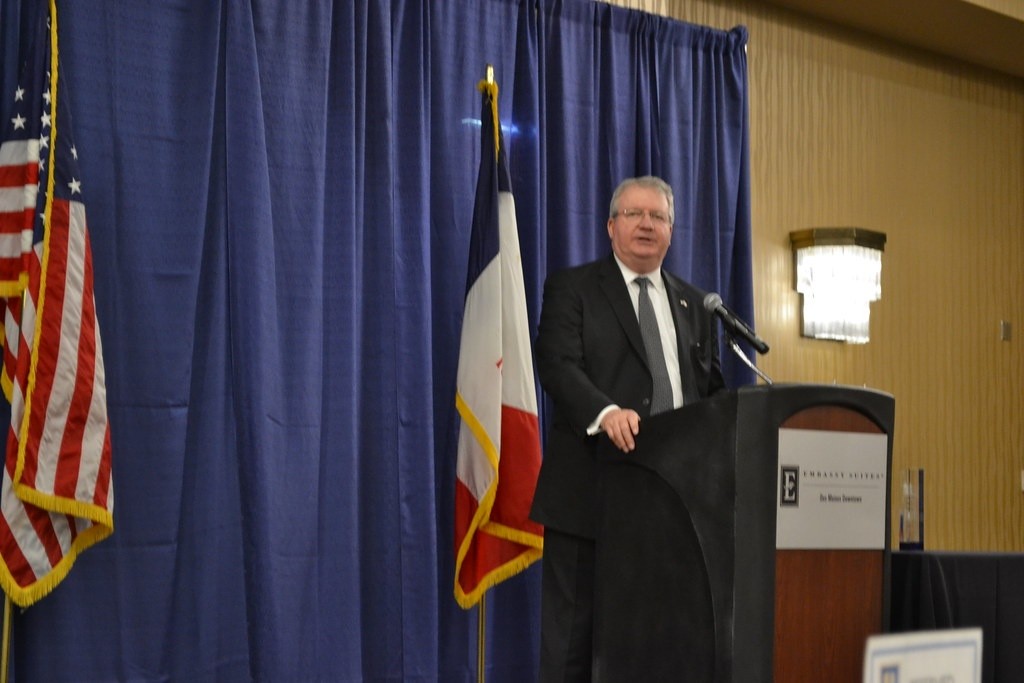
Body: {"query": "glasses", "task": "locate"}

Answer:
[613,208,670,225]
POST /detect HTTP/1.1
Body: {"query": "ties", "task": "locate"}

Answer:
[634,278,674,417]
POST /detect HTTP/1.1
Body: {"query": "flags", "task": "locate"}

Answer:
[0,1,115,606]
[454,79,544,608]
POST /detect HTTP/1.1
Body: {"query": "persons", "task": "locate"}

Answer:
[528,178,725,683]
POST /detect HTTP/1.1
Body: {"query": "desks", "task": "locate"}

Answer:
[887,550,1022,683]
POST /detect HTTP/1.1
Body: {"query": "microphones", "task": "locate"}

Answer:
[703,293,770,355]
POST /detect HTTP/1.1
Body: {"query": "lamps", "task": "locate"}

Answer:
[791,227,888,346]
